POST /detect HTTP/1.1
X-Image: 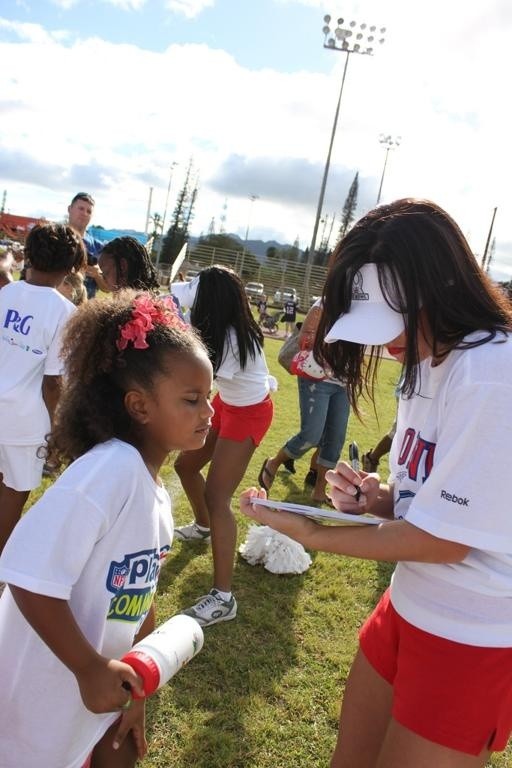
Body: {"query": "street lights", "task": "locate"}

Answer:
[297,8,388,317]
[377,133,402,207]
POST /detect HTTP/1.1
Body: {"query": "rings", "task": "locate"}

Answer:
[121,690,135,709]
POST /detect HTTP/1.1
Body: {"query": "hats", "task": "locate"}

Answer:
[323,263,422,346]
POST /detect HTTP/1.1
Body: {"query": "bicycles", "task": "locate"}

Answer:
[257,309,283,335]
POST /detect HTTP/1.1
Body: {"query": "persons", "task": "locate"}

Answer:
[256,290,362,508]
[0,289,216,768]
[239,199,511,768]
[362,416,398,473]
[1,190,277,628]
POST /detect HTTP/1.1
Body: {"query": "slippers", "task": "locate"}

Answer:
[313,495,335,508]
[258,457,275,491]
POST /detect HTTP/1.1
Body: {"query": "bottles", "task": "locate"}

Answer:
[120,611,206,700]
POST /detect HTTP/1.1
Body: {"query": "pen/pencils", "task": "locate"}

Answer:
[349,440,361,504]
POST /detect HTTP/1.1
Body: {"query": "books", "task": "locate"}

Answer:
[250,490,382,529]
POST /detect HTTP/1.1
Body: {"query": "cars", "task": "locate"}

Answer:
[244,280,320,309]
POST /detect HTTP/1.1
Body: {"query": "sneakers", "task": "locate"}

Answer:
[282,459,296,474]
[363,449,379,471]
[174,521,211,541]
[180,590,237,626]
[305,468,318,487]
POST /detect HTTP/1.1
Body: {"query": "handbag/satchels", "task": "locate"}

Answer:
[291,350,328,383]
[278,323,303,374]
